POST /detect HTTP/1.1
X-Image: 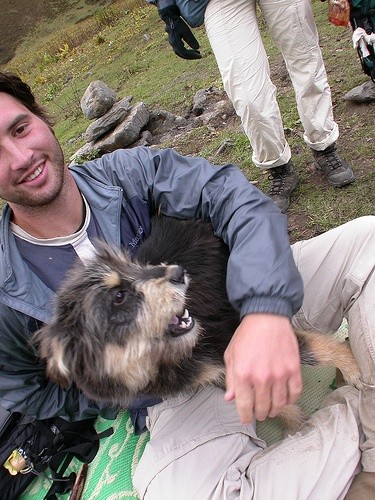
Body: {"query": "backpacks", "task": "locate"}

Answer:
[0,409,115,499]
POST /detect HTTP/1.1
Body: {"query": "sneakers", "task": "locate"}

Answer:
[312,145,355,187]
[266,160,298,213]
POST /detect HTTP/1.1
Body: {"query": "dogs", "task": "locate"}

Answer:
[30,216,363,432]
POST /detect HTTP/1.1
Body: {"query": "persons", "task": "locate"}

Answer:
[1,70,375,500]
[144,0,354,214]
[321,0,375,84]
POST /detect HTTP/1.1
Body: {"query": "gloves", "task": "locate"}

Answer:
[158,5,202,60]
